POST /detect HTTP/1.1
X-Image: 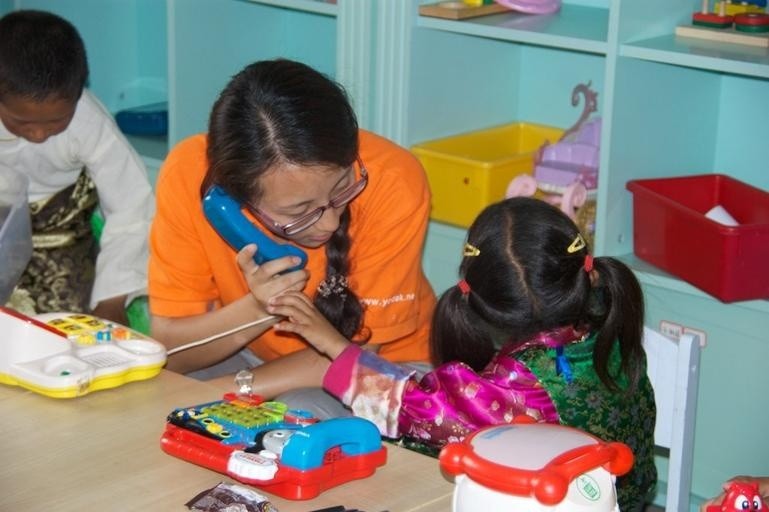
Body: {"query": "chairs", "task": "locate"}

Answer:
[640,325,700,511]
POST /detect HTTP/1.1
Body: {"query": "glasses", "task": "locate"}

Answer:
[235,151,370,238]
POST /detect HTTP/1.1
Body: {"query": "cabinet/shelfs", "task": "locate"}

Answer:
[0,5,767,305]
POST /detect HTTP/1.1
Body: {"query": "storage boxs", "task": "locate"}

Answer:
[627,174,768,303]
[410,124,565,229]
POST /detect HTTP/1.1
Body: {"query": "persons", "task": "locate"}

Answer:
[2,9,155,326]
[264,197,659,510]
[149,60,438,395]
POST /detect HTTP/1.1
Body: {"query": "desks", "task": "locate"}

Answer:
[0,368,457,509]
